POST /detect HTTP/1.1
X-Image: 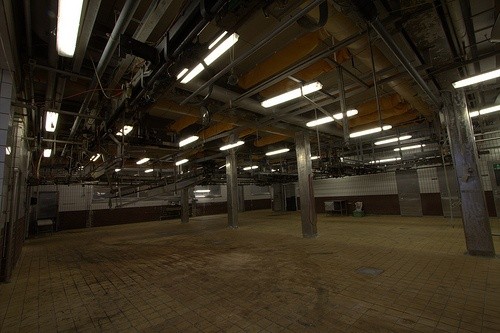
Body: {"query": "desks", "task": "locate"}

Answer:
[324,200,347,217]
